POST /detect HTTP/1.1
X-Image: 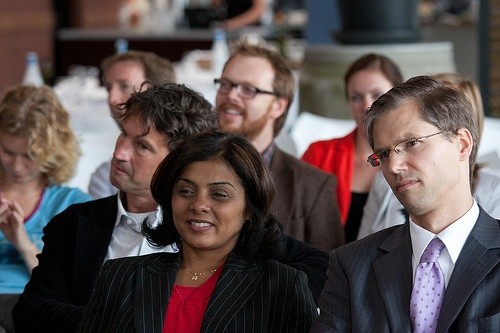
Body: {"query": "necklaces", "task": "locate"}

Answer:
[181,265,219,281]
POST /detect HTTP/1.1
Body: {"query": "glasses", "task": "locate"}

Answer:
[214,78,279,101]
[366,130,446,167]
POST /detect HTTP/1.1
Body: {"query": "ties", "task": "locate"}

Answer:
[408,239,446,333]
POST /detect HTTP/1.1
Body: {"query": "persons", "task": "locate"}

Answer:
[300,52,401,243]
[11,79,330,333]
[0,84,96,333]
[87,50,179,200]
[355,74,500,239]
[214,0,267,28]
[317,74,500,333]
[76,130,320,333]
[212,42,345,252]
